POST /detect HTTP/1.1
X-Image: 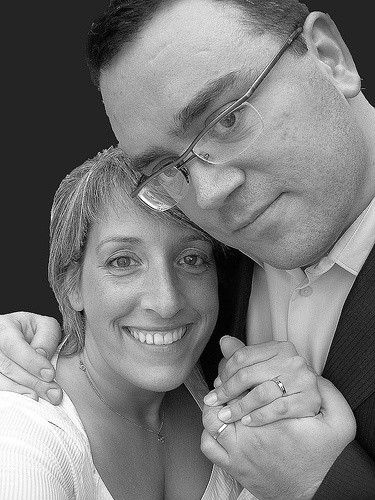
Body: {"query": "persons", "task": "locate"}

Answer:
[0,141,325,500]
[0,0,375,500]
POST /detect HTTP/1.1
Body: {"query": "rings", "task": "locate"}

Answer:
[212,421,231,441]
[268,377,288,398]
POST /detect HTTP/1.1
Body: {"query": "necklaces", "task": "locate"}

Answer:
[75,350,169,446]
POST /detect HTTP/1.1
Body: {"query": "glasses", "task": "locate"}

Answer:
[125,22,312,214]
